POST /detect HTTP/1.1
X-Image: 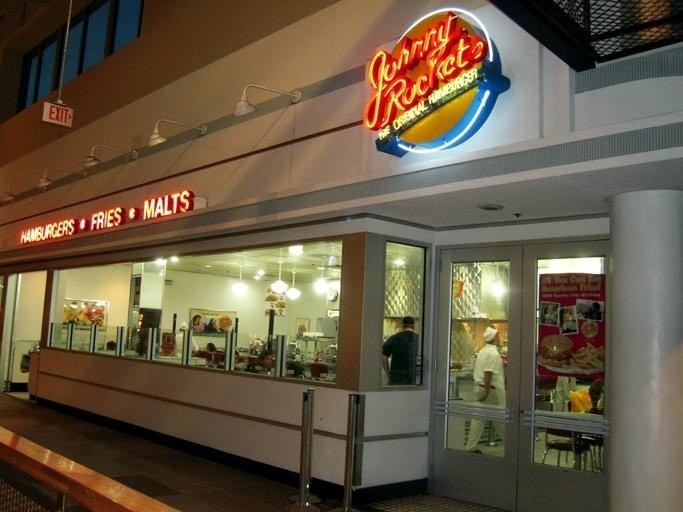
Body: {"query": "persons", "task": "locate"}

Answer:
[174,322,200,357]
[255,349,273,376]
[559,309,577,332]
[464,326,506,456]
[191,314,204,333]
[549,303,559,324]
[589,302,601,319]
[543,304,552,322]
[205,342,217,353]
[382,316,422,385]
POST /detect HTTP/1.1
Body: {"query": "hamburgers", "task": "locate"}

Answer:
[540,335,573,367]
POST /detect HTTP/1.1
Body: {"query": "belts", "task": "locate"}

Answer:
[479,384,495,389]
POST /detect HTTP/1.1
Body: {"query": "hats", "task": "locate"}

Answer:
[400,316,415,324]
[482,326,498,343]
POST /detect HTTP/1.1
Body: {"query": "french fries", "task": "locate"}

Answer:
[571,342,606,369]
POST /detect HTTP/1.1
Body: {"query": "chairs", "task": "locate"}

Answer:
[543,401,604,473]
